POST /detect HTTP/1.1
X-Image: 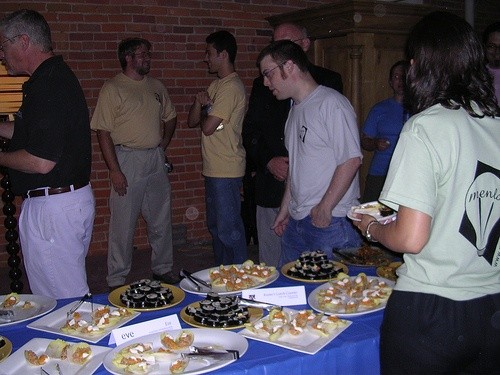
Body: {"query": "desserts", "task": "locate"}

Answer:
[2,293,20,308]
[112,330,195,374]
[23,339,94,366]
[61,304,134,336]
[318,271,393,312]
[208,259,276,290]
[244,308,340,341]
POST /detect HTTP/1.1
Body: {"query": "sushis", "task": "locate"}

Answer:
[287,248,345,280]
[184,292,250,327]
[380,207,394,217]
[121,279,174,308]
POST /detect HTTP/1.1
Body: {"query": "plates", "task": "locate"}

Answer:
[0,335,13,361]
[377,262,404,280]
[108,284,185,312]
[237,307,353,355]
[280,260,349,282]
[347,201,397,221]
[333,247,381,266]
[180,264,280,296]
[0,337,113,375]
[0,294,57,326]
[308,276,395,316]
[180,300,264,329]
[103,328,241,372]
[27,300,141,344]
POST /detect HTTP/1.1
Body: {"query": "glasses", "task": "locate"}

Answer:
[263,60,287,78]
[133,49,153,58]
[391,72,404,79]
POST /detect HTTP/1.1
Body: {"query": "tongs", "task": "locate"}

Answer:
[332,247,367,265]
[235,296,283,311]
[180,268,212,292]
[181,346,240,360]
[66,292,93,318]
[40,363,60,375]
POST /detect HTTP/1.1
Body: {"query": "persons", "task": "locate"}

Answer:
[188,30,248,267]
[481,22,500,108]
[0,8,96,298]
[361,61,406,217]
[90,38,178,292]
[241,22,343,266]
[257,39,364,267]
[354,10,500,375]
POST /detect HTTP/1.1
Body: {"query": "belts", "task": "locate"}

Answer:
[24,182,88,199]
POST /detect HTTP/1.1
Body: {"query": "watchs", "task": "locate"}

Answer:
[366,220,381,241]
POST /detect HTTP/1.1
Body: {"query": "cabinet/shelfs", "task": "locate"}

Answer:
[264,0,446,197]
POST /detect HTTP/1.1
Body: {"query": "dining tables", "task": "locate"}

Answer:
[0,256,401,375]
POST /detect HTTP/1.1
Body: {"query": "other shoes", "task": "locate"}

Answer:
[152,270,179,284]
